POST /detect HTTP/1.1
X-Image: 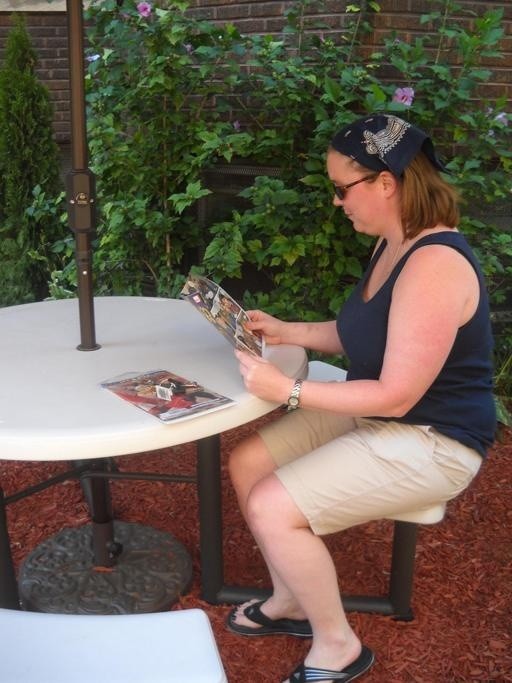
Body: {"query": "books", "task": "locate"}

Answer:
[99,369,237,425]
[180,270,267,361]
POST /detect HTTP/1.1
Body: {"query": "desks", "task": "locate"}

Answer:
[0,295,314,609]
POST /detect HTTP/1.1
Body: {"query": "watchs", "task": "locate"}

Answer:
[287,378,303,411]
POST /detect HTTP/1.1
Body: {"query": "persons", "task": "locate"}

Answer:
[223,114,498,682]
[125,382,214,409]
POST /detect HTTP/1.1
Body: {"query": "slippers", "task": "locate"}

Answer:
[225,598,313,638]
[277,647,374,682]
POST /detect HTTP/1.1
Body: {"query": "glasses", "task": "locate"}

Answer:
[332,174,371,199]
